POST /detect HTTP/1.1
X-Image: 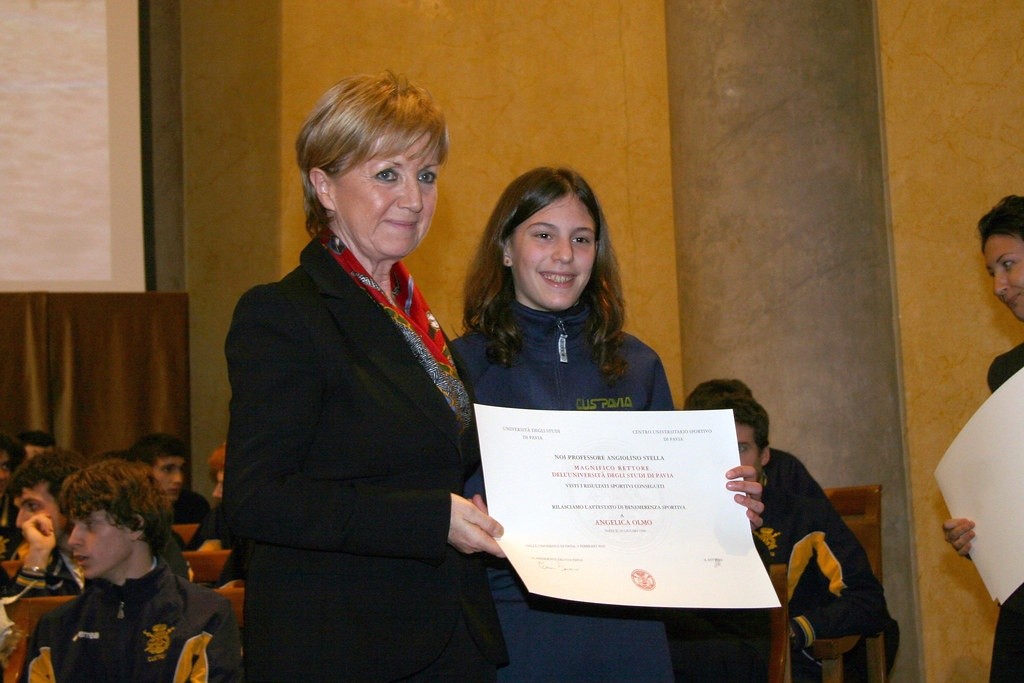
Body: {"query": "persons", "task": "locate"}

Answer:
[17,458,246,683]
[0,433,27,561]
[186,441,231,552]
[684,379,827,500]
[943,193,1023,683]
[10,450,193,597]
[222,70,510,683]
[20,431,55,462]
[680,378,900,682]
[448,166,766,682]
[128,432,211,523]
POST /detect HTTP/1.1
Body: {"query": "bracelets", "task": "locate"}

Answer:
[21,565,49,575]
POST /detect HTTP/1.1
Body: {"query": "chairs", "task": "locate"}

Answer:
[0,484,889,683]
